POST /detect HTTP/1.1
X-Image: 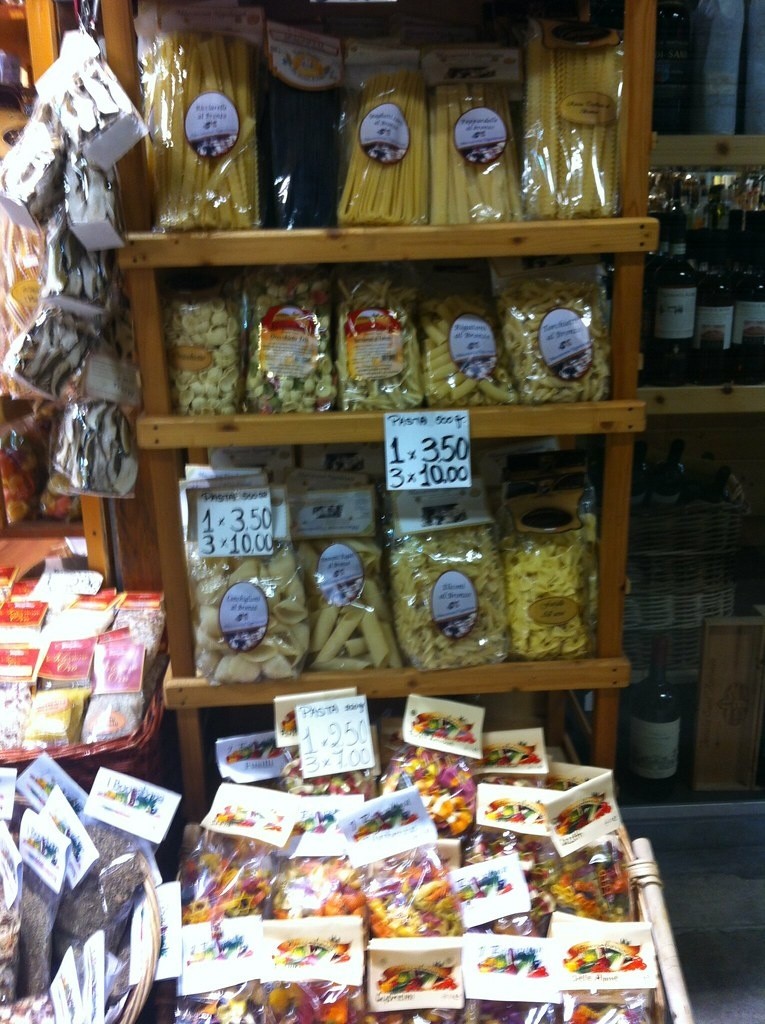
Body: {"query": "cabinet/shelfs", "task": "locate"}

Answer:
[0,0,158,799]
[99,0,655,862]
[620,133,765,820]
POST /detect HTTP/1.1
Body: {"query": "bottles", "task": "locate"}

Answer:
[694,465,732,503]
[637,171,765,387]
[652,0,765,136]
[628,634,682,800]
[630,440,648,506]
[649,439,686,504]
